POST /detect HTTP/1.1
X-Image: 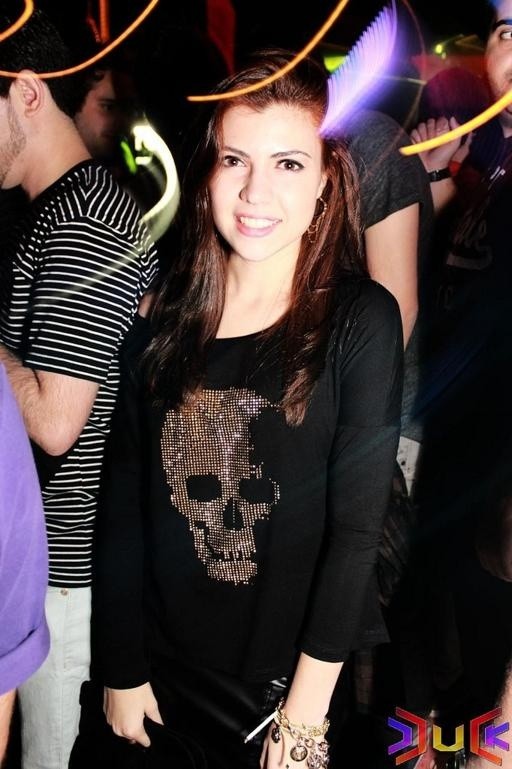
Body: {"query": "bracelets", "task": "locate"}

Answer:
[425,167,454,181]
[272,697,332,768]
[445,159,463,175]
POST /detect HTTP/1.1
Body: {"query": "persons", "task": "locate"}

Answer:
[0,38,162,769]
[446,129,472,179]
[0,367,50,767]
[407,110,474,212]
[51,60,136,158]
[321,107,427,620]
[369,0,512,767]
[67,50,403,769]
